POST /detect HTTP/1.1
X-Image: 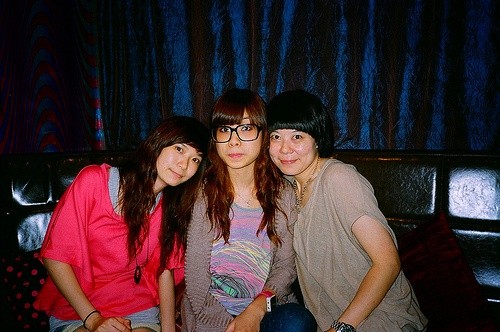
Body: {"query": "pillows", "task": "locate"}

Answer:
[0,247,52,332]
[394,211,497,332]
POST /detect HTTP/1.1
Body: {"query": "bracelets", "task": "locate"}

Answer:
[83,310,101,329]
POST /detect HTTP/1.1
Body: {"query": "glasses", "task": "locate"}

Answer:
[211,123,262,143]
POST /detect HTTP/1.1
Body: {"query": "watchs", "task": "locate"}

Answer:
[331,319,357,332]
[256,290,277,312]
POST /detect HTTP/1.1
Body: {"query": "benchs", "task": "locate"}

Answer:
[0,148,500,332]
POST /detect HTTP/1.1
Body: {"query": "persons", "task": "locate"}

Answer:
[32,116,210,332]
[267,90,429,332]
[182,88,317,332]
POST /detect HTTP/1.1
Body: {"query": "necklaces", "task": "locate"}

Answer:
[293,157,319,214]
[133,254,148,285]
